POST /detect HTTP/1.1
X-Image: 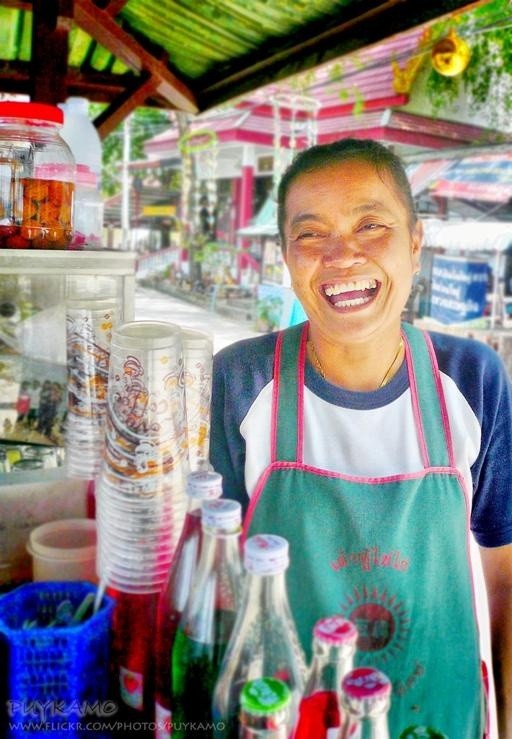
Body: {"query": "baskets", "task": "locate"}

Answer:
[0,580,115,739]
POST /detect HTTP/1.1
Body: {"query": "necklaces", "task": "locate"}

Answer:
[311,341,404,393]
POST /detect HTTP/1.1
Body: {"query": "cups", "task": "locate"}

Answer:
[65,294,212,594]
[26,519,100,584]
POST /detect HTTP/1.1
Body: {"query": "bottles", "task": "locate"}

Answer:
[35,162,107,250]
[57,93,103,179]
[153,469,222,739]
[106,583,164,739]
[170,499,244,738]
[209,533,308,739]
[295,615,358,739]
[238,677,292,739]
[0,100,74,250]
[341,667,391,739]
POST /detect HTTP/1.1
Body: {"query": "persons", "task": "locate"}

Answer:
[211,139,512,739]
